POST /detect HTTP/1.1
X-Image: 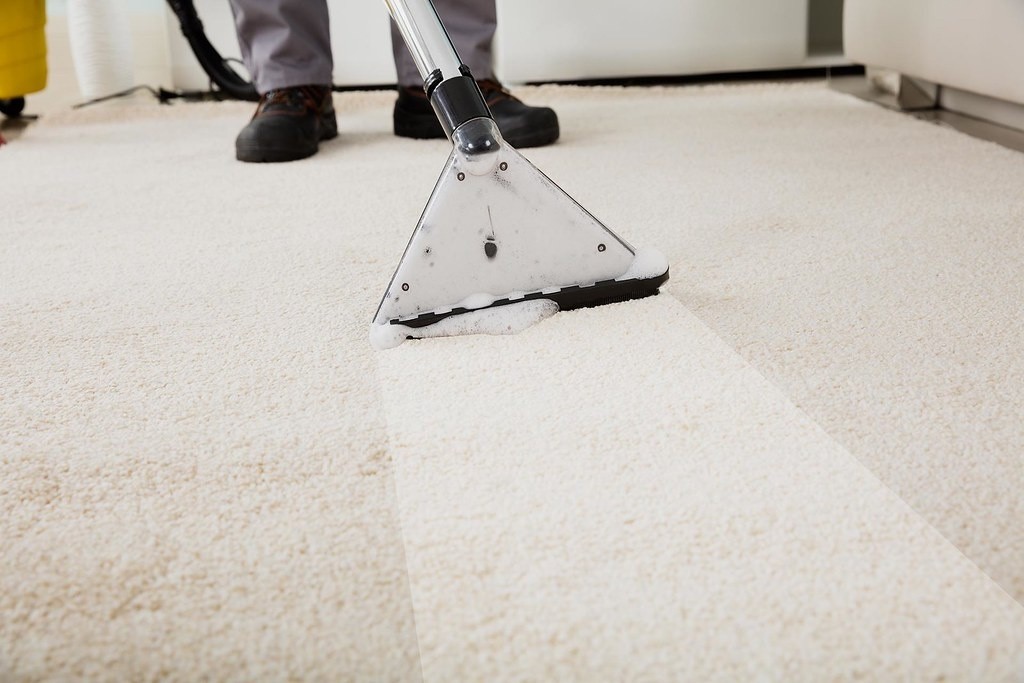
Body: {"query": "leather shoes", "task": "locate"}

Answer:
[394,79,559,150]
[236,81,340,163]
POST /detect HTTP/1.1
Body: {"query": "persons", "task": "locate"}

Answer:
[226,0,559,162]
